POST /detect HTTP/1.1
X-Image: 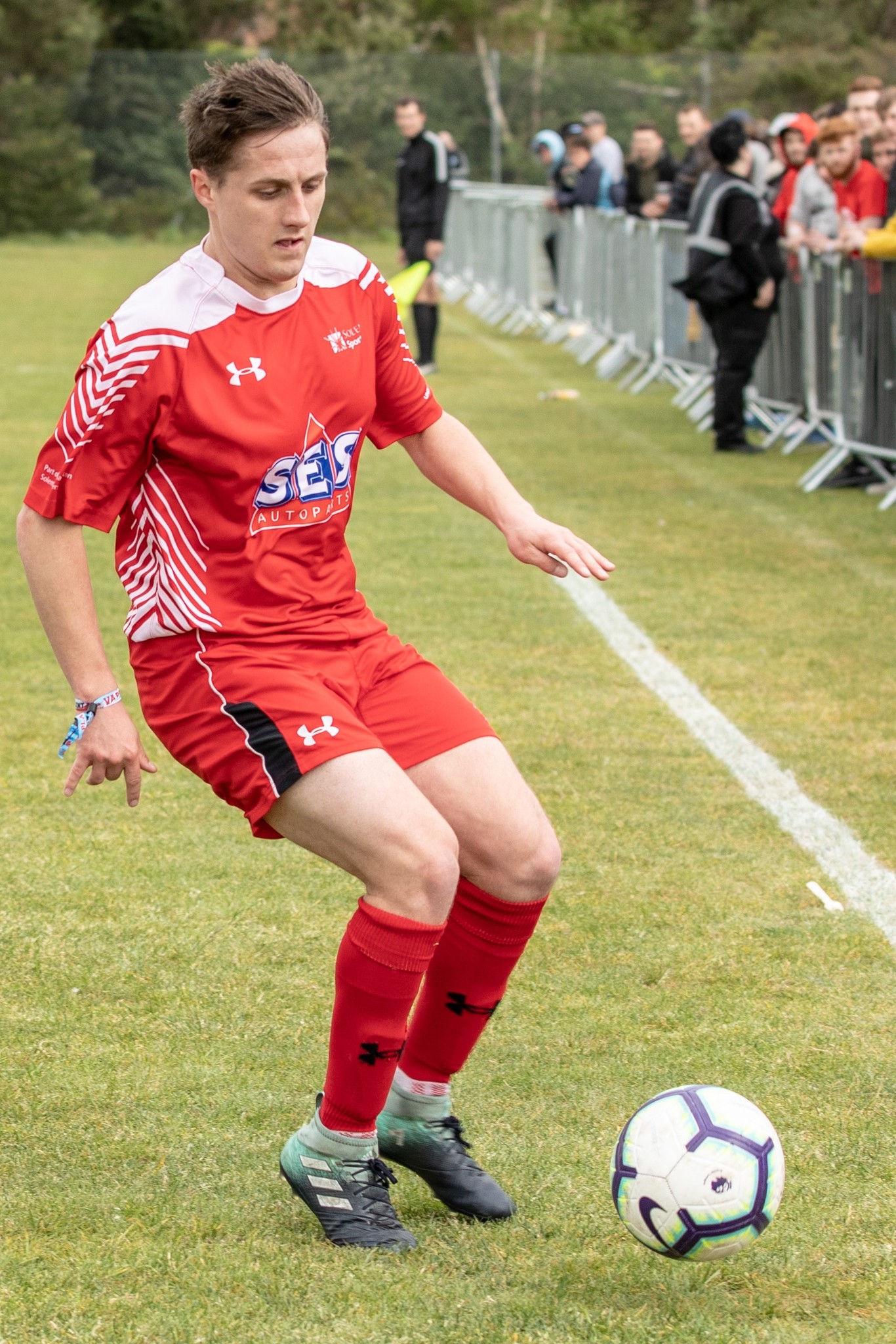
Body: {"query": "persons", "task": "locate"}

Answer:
[673,116,781,455]
[534,72,896,462]
[395,98,449,374]
[15,57,616,1248]
[439,130,469,184]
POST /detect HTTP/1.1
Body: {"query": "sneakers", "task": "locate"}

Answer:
[376,1109,518,1223]
[279,1091,419,1252]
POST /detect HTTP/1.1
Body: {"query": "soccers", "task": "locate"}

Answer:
[609,1084,785,1262]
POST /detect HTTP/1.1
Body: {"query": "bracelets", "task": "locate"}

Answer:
[56,687,123,761]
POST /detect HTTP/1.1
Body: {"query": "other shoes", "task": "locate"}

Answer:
[834,458,895,488]
[416,359,436,376]
[715,439,765,454]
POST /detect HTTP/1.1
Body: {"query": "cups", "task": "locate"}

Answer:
[654,180,671,196]
